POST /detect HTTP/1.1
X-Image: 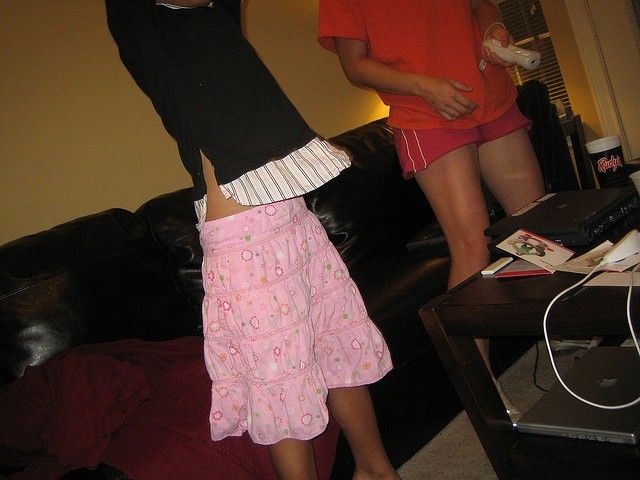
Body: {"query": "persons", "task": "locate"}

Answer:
[510,243,535,256]
[525,241,546,257]
[317,1,546,426]
[106,0,394,480]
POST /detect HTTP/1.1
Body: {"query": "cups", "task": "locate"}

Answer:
[586,135,628,190]
[630,170,640,195]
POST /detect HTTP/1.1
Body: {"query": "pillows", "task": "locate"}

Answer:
[154,197,367,337]
[0,269,72,382]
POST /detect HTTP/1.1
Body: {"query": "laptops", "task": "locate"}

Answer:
[516,346,640,444]
[483,190,639,248]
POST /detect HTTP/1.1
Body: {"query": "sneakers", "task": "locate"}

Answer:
[493,378,524,427]
[551,339,591,353]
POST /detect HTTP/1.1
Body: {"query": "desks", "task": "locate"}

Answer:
[563,114,596,190]
[420,188,640,479]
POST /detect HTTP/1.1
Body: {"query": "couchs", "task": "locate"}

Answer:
[0,77,582,480]
